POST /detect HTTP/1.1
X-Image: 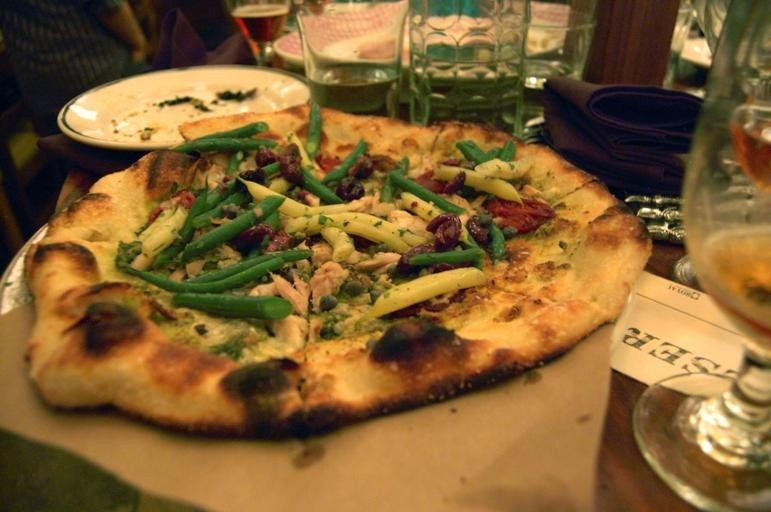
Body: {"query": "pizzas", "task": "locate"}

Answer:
[24,102,655,437]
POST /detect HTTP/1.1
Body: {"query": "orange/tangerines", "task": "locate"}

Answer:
[358,28,399,59]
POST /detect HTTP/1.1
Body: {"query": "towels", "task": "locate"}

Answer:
[542,72,707,197]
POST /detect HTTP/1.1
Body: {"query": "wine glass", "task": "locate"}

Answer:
[630,0,771,512]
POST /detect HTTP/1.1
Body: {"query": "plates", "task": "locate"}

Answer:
[51,63,313,155]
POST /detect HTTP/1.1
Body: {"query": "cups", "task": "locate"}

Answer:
[226,0,598,141]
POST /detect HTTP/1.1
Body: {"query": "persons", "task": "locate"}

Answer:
[0,0,147,181]
